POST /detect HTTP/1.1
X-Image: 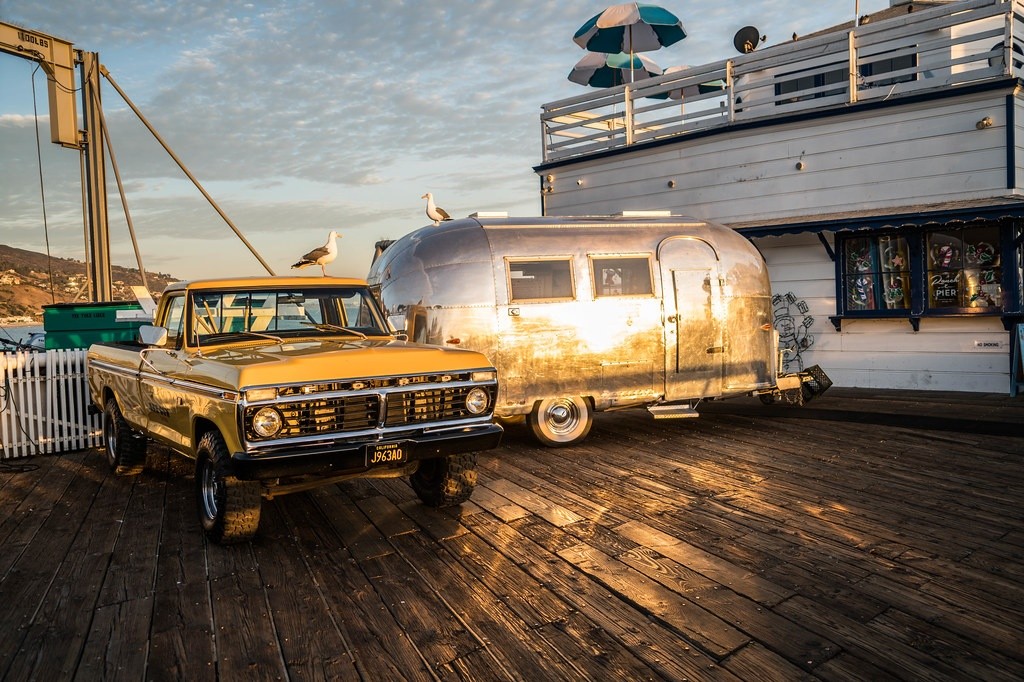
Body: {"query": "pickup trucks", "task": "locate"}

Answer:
[87,278,504,545]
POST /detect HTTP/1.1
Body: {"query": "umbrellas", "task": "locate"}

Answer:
[566,50,664,149]
[660,64,726,126]
[572,2,688,145]
